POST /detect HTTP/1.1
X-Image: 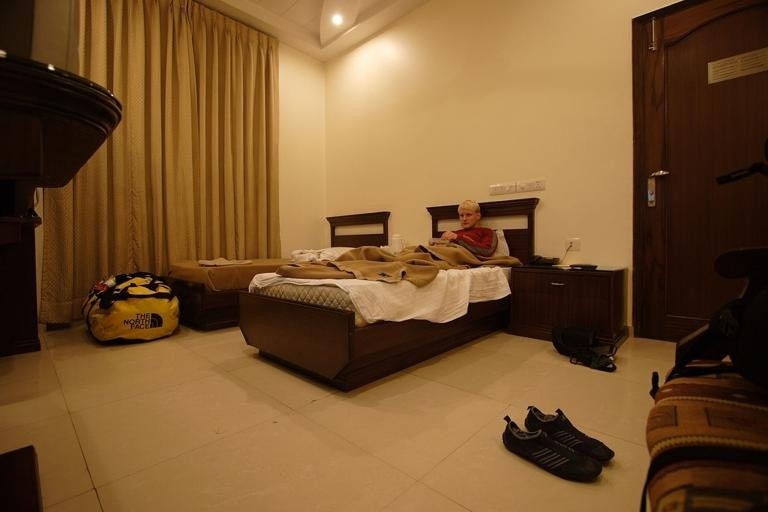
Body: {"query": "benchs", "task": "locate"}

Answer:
[641,242,768,511]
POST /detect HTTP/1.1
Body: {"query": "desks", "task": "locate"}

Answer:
[1,51,126,359]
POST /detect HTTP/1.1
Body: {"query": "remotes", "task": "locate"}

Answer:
[569,263,598,270]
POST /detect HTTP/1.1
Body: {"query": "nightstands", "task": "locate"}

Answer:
[508,263,629,356]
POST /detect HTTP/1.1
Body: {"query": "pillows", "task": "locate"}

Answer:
[492,227,511,258]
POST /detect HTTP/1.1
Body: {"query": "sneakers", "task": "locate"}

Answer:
[502,405,615,482]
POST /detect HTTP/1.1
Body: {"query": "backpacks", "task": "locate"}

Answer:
[551,321,616,375]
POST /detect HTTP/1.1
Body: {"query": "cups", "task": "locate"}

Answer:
[391,235,405,255]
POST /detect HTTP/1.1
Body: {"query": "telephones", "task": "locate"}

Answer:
[529,255,560,266]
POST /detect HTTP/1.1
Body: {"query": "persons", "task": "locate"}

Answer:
[441,199,498,256]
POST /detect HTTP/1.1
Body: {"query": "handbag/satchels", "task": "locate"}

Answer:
[82,269,183,344]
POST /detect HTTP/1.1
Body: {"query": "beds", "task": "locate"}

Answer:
[168,209,391,333]
[237,197,541,394]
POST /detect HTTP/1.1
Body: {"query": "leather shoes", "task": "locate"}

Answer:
[1,54,123,188]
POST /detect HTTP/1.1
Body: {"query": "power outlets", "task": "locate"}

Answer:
[534,179,545,191]
[565,238,581,252]
